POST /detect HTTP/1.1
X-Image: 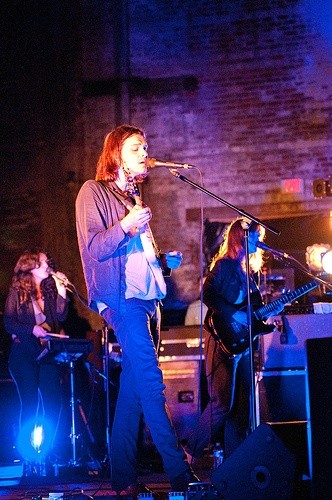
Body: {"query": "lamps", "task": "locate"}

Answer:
[305,243,332,274]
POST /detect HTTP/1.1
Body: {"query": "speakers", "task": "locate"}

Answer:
[209,427,302,500]
[144,361,202,451]
[0,380,25,467]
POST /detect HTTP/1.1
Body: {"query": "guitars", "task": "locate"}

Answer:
[122,166,172,278]
[208,271,330,360]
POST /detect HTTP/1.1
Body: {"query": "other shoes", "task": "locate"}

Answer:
[169,469,215,490]
[114,479,153,494]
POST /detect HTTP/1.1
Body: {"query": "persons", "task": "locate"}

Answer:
[198,217,282,459]
[4,248,69,464]
[75,124,204,493]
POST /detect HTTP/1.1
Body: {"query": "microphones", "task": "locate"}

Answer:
[255,241,294,260]
[144,157,196,169]
[46,267,73,285]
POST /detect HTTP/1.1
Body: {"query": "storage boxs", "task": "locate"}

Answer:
[254,371,312,480]
